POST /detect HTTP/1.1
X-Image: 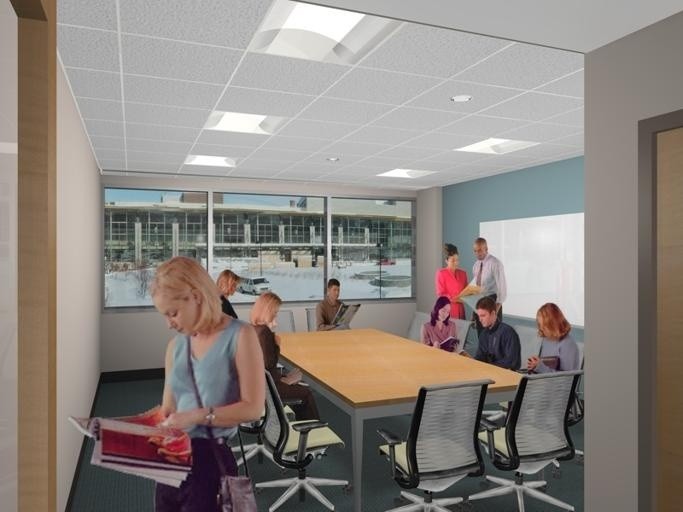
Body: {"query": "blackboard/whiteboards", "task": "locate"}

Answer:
[478,210,587,330]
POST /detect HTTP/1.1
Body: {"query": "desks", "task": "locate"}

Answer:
[274,328,524,510]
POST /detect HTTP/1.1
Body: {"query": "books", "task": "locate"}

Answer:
[333,302,363,325]
[68,405,193,487]
[440,337,460,349]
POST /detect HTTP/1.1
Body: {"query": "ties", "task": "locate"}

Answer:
[476,261,484,287]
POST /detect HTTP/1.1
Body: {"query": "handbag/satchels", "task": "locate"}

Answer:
[564,392,584,426]
[216,474,258,512]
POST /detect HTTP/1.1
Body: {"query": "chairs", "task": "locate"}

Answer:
[467,370,582,511]
[306,308,316,331]
[231,368,352,512]
[376,380,496,512]
[274,310,296,332]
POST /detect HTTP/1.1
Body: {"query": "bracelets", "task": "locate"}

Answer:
[206,406,214,426]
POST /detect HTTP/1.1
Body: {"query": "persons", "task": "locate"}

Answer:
[423,297,456,352]
[215,269,241,320]
[436,243,468,320]
[252,291,319,423]
[316,278,346,330]
[465,296,520,370]
[150,255,266,512]
[528,302,581,373]
[472,238,506,338]
